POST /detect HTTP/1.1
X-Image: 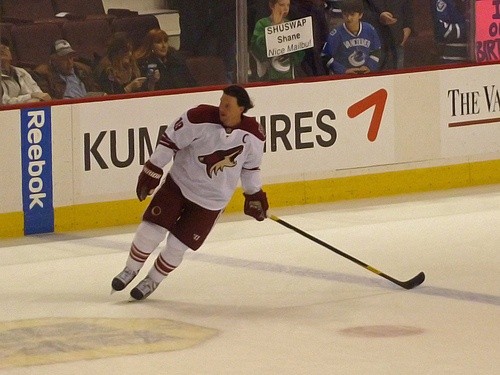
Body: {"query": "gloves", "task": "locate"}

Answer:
[243,190,268,221]
[137,160,163,200]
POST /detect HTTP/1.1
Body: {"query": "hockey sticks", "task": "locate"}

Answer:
[265,211,426,291]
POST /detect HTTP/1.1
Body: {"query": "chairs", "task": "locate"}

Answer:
[0,0,229,99]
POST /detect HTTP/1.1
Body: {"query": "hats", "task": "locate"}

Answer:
[52,39,76,56]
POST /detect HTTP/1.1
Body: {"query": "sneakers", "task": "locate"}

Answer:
[129,276,159,302]
[110,267,139,295]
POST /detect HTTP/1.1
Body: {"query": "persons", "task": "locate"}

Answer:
[432,0,469,65]
[112,85,269,301]
[251,0,412,83]
[0,29,198,106]
